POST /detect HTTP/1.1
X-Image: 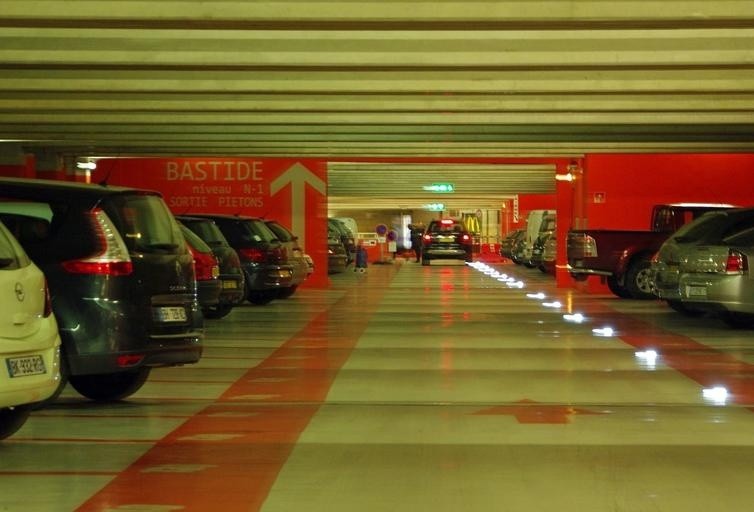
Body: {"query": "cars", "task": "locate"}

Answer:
[500,209,556,272]
[652,208,753,329]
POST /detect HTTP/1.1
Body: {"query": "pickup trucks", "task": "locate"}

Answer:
[566,203,740,300]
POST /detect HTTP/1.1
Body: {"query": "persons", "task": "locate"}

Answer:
[408,224,422,263]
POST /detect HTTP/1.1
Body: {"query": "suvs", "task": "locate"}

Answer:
[418,218,472,266]
